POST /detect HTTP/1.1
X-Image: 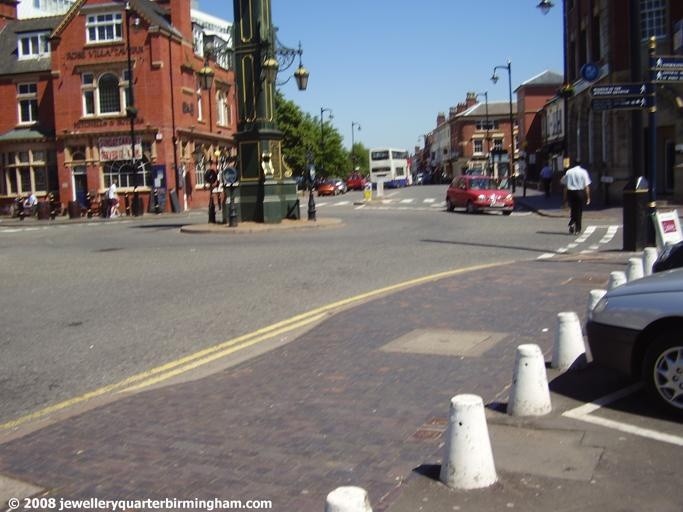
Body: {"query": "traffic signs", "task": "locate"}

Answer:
[650,71,683,83]
[650,55,682,70]
[589,84,651,98]
[592,95,649,114]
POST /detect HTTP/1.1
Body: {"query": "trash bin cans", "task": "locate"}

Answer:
[623,176,650,252]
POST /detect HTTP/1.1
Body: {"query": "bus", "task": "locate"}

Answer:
[369,147,410,189]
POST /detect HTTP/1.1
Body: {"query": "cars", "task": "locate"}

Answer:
[586,241,682,421]
[445,175,515,216]
[318,173,367,196]
[415,173,424,185]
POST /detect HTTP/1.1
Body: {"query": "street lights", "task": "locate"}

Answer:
[538,0,653,250]
[351,121,360,171]
[319,106,334,168]
[491,62,515,193]
[473,92,492,175]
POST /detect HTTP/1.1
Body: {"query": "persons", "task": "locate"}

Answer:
[107,179,121,219]
[22,194,38,208]
[558,157,592,237]
[539,162,553,200]
